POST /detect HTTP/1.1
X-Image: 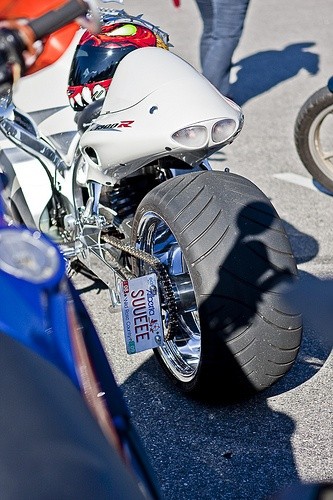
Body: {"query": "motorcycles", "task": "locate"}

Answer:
[0,0,305,404]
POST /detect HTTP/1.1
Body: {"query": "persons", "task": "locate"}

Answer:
[194,0,249,100]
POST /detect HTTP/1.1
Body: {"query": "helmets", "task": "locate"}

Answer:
[67,16,169,122]
